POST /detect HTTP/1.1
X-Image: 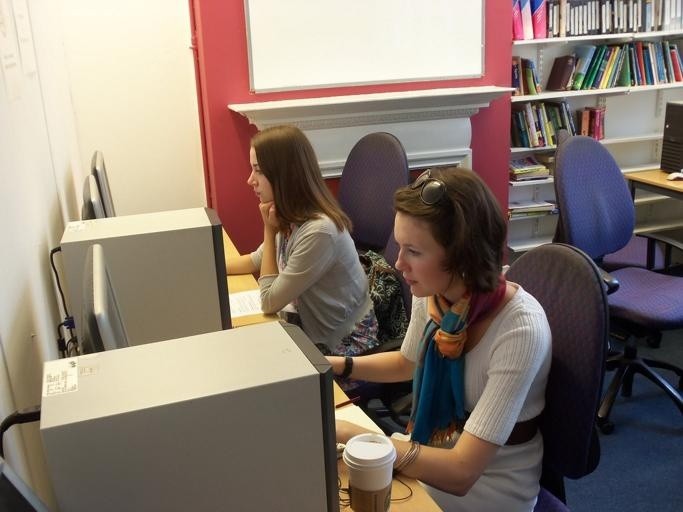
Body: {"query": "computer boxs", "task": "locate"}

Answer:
[60,207,232,354]
[40,320,338,512]
[659,102,683,173]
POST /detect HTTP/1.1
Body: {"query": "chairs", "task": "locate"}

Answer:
[383,225,413,318]
[554,135,683,434]
[502,244,609,510]
[338,132,408,255]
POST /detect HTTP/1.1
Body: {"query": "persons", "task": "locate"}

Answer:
[226,126,379,359]
[324,169,553,507]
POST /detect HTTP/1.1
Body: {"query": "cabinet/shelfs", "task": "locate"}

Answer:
[508,0,683,252]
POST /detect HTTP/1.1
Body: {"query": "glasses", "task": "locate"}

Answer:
[411,169,447,206]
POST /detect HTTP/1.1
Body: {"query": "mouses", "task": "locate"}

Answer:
[666,172,683,181]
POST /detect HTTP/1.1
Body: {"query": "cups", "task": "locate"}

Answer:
[341,433,397,512]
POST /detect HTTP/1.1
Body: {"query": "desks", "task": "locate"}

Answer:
[623,167,683,201]
[222,225,442,511]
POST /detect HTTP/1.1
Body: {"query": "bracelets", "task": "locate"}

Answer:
[340,355,354,381]
[394,439,421,474]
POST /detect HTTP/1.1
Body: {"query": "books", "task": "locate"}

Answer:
[513,0,682,40]
[511,102,602,147]
[547,40,683,91]
[512,56,540,96]
[509,154,557,180]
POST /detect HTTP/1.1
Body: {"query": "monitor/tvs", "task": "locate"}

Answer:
[80,242,131,355]
[81,175,105,221]
[91,150,115,217]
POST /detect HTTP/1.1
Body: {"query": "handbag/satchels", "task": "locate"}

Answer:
[355,249,409,340]
